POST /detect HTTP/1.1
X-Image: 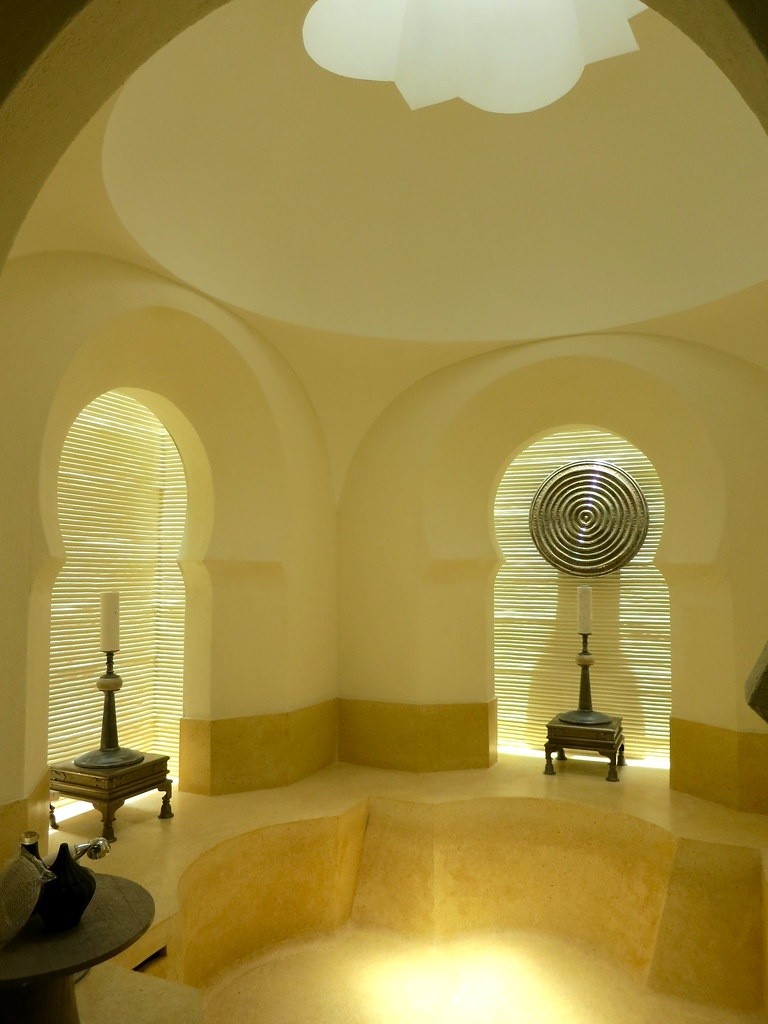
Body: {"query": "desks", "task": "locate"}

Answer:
[47,750,171,846]
[0,872,153,1023]
[542,712,626,781]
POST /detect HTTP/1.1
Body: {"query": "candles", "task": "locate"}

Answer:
[99,590,118,650]
[576,586,592,633]
